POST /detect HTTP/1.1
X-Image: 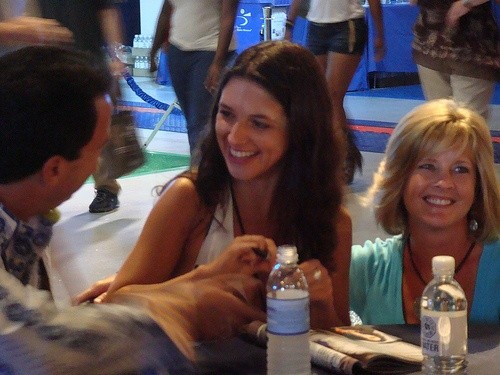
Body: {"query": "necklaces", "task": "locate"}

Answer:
[407,231,477,287]
[230,184,248,237]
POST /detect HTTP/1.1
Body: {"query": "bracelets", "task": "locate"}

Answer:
[463,1,474,9]
[286,20,293,30]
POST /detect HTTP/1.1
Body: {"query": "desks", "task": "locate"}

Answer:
[346,3,424,91]
[193,324,500,375]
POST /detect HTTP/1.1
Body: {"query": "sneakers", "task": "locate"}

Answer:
[87,185,121,213]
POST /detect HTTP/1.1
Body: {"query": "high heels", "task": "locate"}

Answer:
[342,145,367,183]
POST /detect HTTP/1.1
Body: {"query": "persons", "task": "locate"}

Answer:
[108,41,353,331]
[77,98,500,326]
[0,44,277,375]
[1,0,500,213]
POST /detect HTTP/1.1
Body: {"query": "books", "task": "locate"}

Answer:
[256,324,424,375]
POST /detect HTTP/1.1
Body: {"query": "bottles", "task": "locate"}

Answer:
[134,56,148,70]
[264,244,311,375]
[419,255,468,375]
[132,34,155,48]
